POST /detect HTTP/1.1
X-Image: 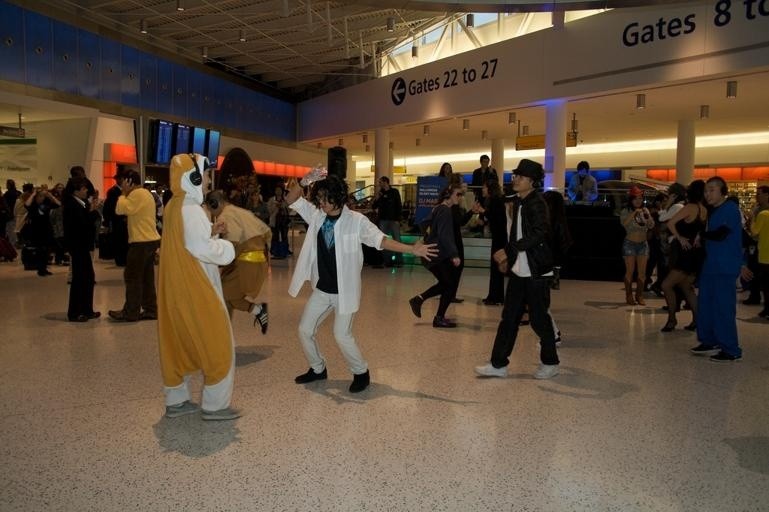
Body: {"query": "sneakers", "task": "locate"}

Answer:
[483,298,504,305]
[165,401,201,417]
[254,303,268,334]
[534,365,559,378]
[68,311,100,321]
[474,363,507,376]
[201,407,241,420]
[538,331,561,345]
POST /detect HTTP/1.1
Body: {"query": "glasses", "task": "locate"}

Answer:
[456,192,462,196]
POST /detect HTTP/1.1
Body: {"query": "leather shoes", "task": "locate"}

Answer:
[627,278,769,362]
[433,316,456,328]
[451,298,463,303]
[38,270,52,276]
[109,310,157,321]
[350,368,369,392]
[295,368,327,383]
[409,296,423,317]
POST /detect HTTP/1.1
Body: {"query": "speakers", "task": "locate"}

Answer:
[327,146,346,178]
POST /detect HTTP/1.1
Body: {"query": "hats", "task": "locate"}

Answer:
[627,186,645,196]
[205,159,217,170]
[512,159,545,178]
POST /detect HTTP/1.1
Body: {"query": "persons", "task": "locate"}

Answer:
[202,173,294,335]
[473,160,559,378]
[157,154,246,419]
[286,166,439,393]
[1,166,169,322]
[620,176,768,360]
[568,161,598,205]
[410,154,563,347]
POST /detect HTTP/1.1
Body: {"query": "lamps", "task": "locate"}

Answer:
[240,30,247,42]
[365,144,370,150]
[727,82,737,100]
[415,138,422,147]
[521,125,529,136]
[422,123,431,138]
[481,129,488,144]
[202,46,208,57]
[387,17,395,32]
[700,105,711,122]
[176,0,184,11]
[389,140,395,151]
[362,132,368,142]
[412,47,418,56]
[462,118,472,133]
[636,91,646,111]
[318,142,324,149]
[140,17,148,34]
[338,136,345,147]
[466,14,473,28]
[508,110,515,126]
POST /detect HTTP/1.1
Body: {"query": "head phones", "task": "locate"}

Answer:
[331,174,348,205]
[634,208,649,226]
[532,180,543,188]
[188,153,202,185]
[208,199,218,210]
[721,185,728,195]
[443,192,451,200]
[126,169,133,186]
